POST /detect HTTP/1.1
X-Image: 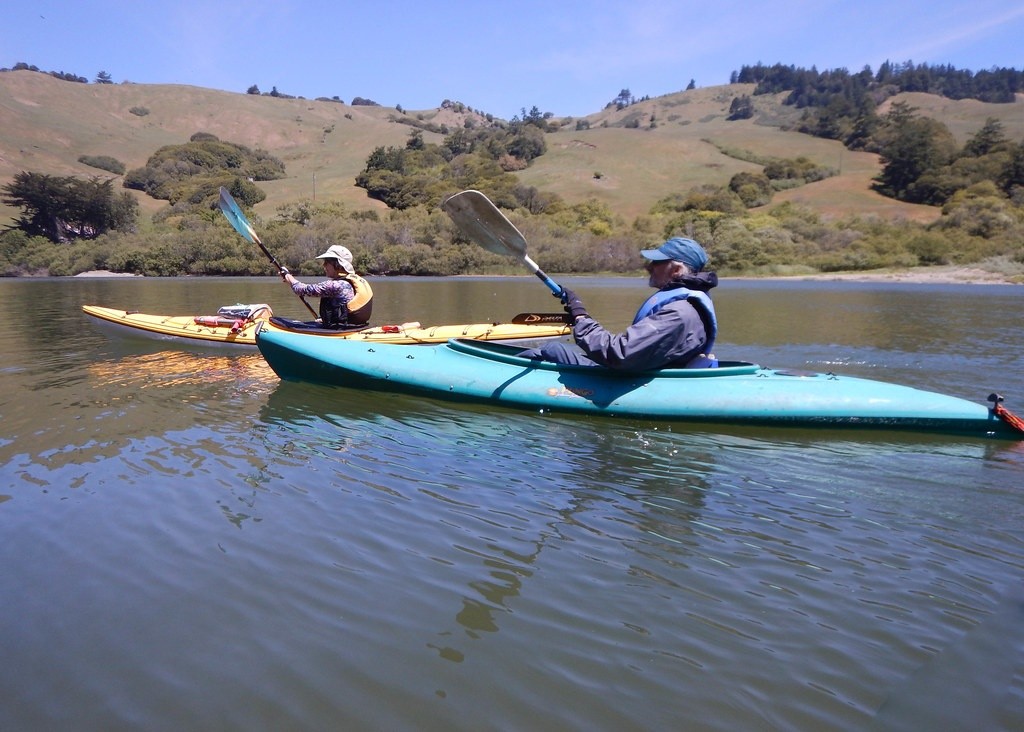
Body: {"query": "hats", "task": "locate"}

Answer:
[314,244,356,273]
[640,236,707,273]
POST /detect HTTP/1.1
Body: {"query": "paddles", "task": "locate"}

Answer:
[446,188,566,298]
[214,186,319,319]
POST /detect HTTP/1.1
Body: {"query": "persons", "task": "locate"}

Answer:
[277,245,373,325]
[513,237,718,372]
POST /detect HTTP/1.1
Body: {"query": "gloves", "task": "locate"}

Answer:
[553,285,589,322]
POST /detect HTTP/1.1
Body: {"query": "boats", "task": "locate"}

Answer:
[82,304,574,352]
[256,326,1024,431]
